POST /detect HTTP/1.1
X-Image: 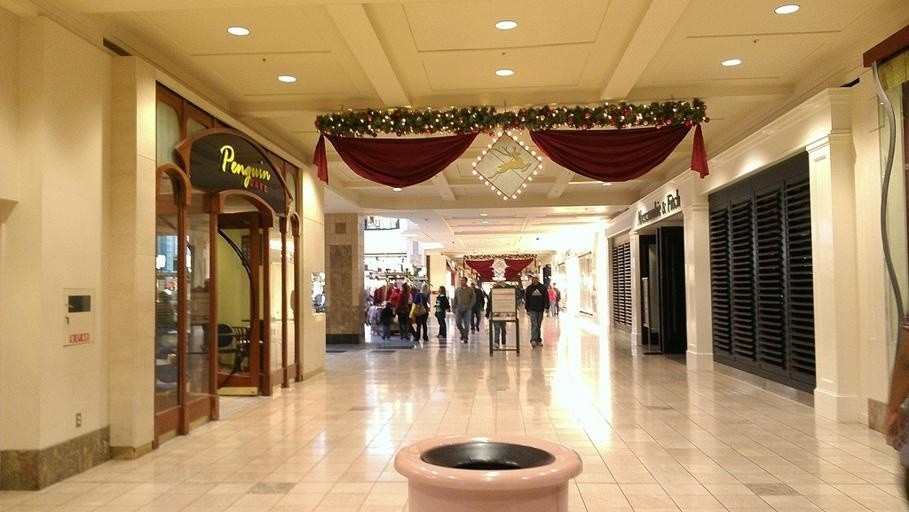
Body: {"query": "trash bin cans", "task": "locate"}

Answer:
[394,434,583,512]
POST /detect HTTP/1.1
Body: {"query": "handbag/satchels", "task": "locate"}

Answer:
[409,293,427,320]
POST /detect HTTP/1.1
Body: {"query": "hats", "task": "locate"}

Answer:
[528,273,539,278]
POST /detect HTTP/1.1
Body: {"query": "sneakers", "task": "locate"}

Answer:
[381,334,507,347]
[530,340,543,349]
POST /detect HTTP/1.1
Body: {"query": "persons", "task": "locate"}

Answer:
[544,280,561,316]
[516,285,525,306]
[314,290,326,307]
[452,276,476,343]
[476,284,489,311]
[365,282,430,344]
[485,276,510,348]
[431,285,450,338]
[524,272,550,348]
[470,283,485,334]
